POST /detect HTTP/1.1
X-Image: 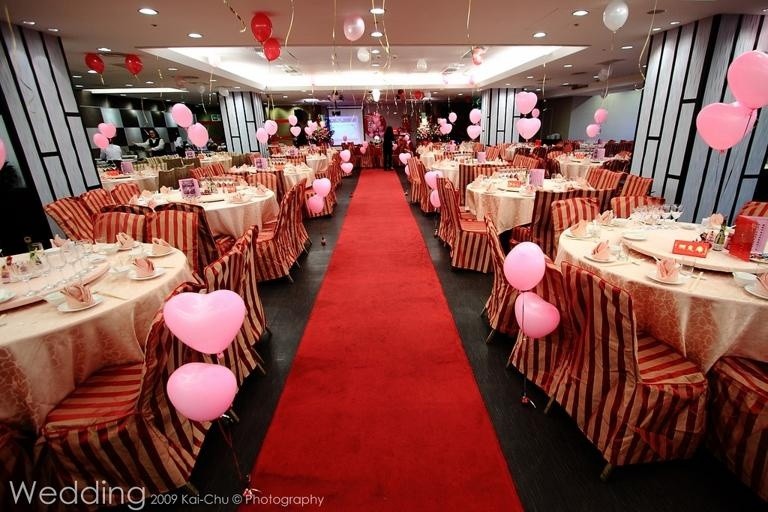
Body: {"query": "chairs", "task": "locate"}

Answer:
[306,138,449,218]
[435,139,768,508]
[0,137,312,512]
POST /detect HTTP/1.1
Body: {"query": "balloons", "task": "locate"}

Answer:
[85,12,542,215]
[0,137,7,171]
[503,242,545,291]
[696,102,758,154]
[728,51,768,109]
[515,292,560,338]
[167,362,237,422]
[163,290,246,355]
[586,124,599,137]
[603,0,629,32]
[594,108,608,125]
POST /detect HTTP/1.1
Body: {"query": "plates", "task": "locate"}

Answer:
[563,221,768,300]
[58,295,102,313]
[133,185,272,210]
[0,240,173,305]
[229,164,309,174]
[465,174,582,196]
[559,150,618,163]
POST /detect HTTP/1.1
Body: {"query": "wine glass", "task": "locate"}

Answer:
[98,164,117,172]
[632,203,685,230]
[199,175,234,194]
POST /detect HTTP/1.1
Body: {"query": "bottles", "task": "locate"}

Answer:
[712,214,730,251]
